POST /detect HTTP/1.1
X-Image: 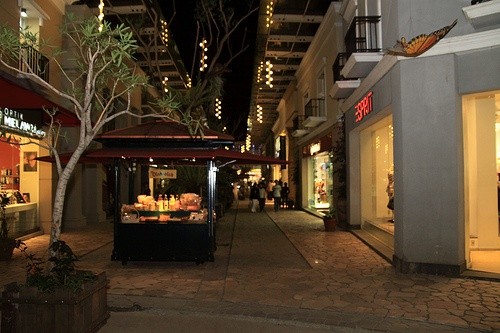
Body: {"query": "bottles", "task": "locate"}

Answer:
[175,194,180,210]
[157,194,164,211]
[163,194,169,211]
[169,194,175,210]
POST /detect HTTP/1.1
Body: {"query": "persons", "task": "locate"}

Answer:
[249,179,291,214]
[24,152,37,171]
[386,172,393,223]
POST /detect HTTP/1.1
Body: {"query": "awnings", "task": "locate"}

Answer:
[0,77,96,128]
